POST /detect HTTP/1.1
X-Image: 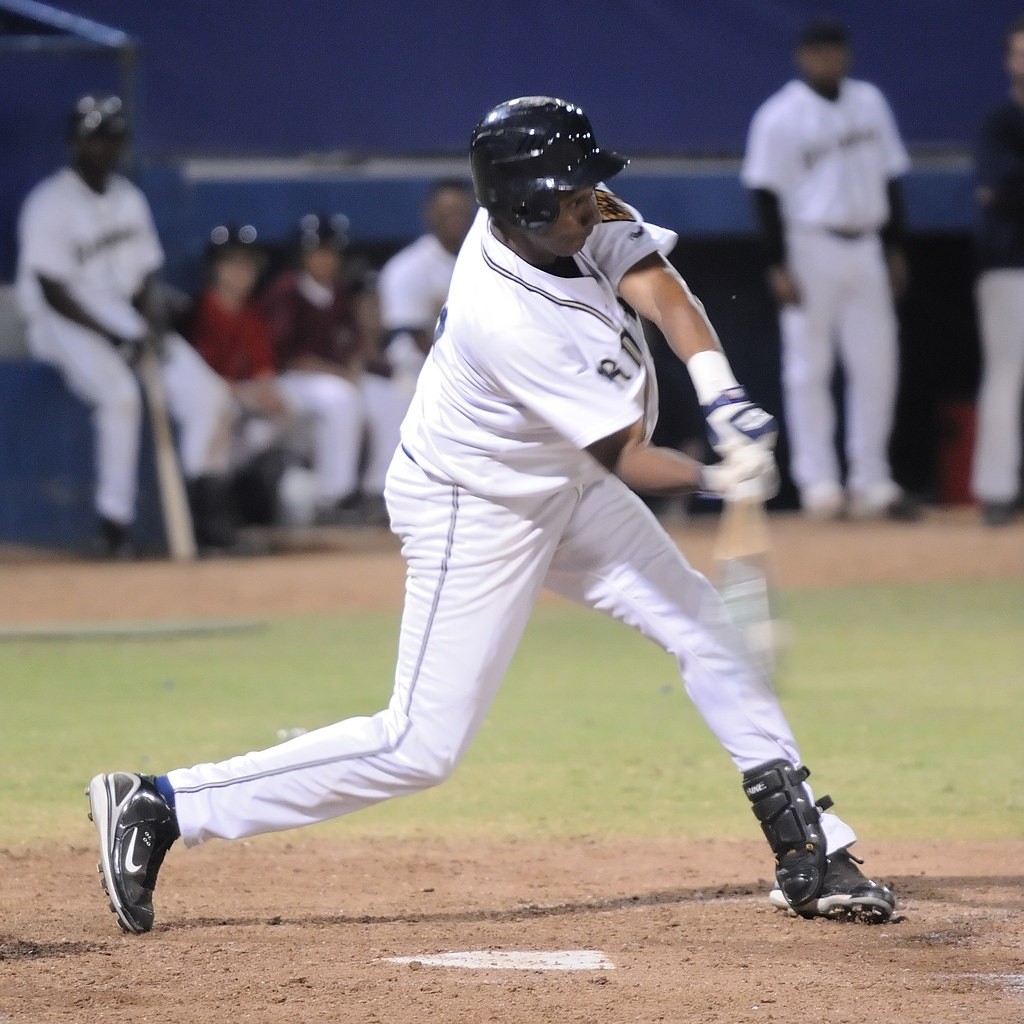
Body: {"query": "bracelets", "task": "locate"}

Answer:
[684,349,745,407]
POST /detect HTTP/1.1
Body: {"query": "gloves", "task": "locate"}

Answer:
[701,444,780,504]
[698,385,779,457]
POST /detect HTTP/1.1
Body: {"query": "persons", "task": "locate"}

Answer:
[736,21,913,521]
[14,100,475,557]
[974,22,1024,522]
[81,97,898,935]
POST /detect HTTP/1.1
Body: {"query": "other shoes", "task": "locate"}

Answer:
[95,516,134,561]
[980,501,1016,525]
[184,478,236,546]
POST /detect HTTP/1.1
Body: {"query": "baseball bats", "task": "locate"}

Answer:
[139,353,199,560]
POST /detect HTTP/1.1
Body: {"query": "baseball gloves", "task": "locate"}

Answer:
[714,447,790,697]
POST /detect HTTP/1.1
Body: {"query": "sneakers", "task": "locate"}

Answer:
[84,772,181,936]
[769,848,895,921]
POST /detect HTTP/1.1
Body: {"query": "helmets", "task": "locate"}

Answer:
[470,95,631,237]
